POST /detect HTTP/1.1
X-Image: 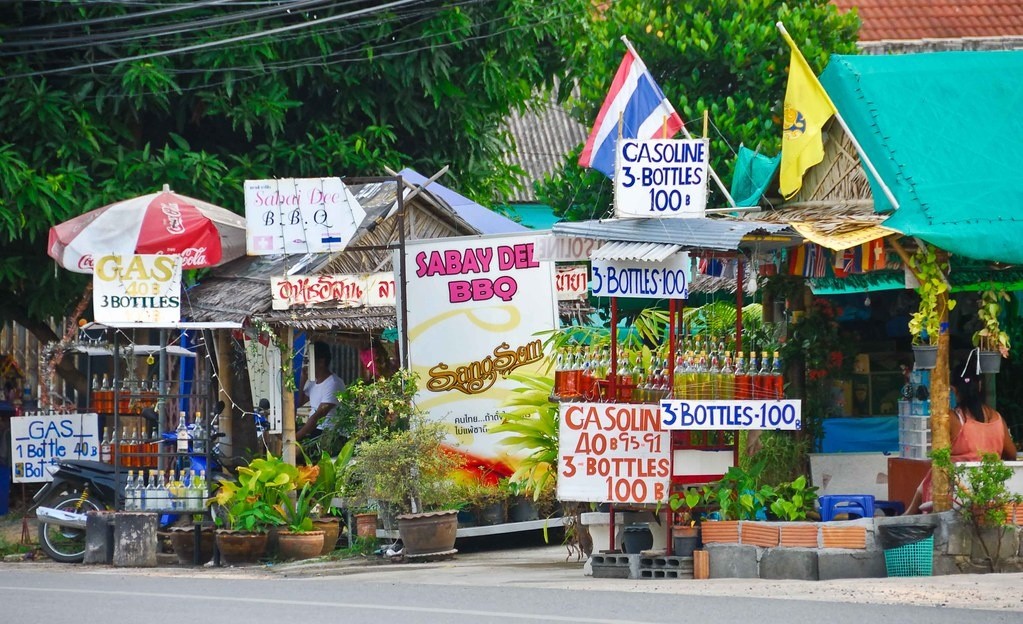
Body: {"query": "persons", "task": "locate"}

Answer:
[949,362,1017,463]
[296,340,349,456]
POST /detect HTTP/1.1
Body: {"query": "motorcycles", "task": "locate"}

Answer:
[212,398,272,469]
[26,400,237,563]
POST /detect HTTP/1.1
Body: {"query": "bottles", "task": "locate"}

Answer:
[90,374,101,413]
[134,470,146,511]
[100,426,110,464]
[139,426,151,468]
[110,427,116,465]
[125,470,136,510]
[145,469,158,512]
[166,470,178,510]
[130,426,140,467]
[120,425,130,467]
[177,411,188,454]
[551,337,784,406]
[178,470,186,510]
[150,379,159,407]
[111,376,121,413]
[128,380,141,414]
[196,468,209,510]
[156,470,166,513]
[100,373,114,413]
[192,411,204,453]
[139,379,151,408]
[186,470,196,511]
[150,427,159,469]
[120,377,131,413]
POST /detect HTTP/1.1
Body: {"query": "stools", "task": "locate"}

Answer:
[816,494,906,523]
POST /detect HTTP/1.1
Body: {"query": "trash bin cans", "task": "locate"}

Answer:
[878,523,938,577]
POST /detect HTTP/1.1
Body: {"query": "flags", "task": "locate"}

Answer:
[578,43,684,183]
[777,36,838,194]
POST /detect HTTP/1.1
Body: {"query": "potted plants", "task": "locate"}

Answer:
[972,290,1012,376]
[905,247,941,373]
[170,413,701,577]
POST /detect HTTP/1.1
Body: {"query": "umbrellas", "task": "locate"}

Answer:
[47,184,246,467]
